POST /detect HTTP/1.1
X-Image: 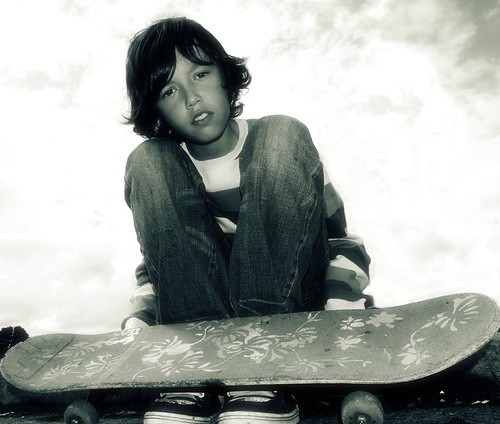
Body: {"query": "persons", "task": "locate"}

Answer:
[119,15,371,424]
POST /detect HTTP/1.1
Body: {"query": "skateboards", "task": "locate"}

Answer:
[0,293,500,424]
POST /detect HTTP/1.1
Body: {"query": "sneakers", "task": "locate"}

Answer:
[216,385,300,423]
[144,387,222,424]
